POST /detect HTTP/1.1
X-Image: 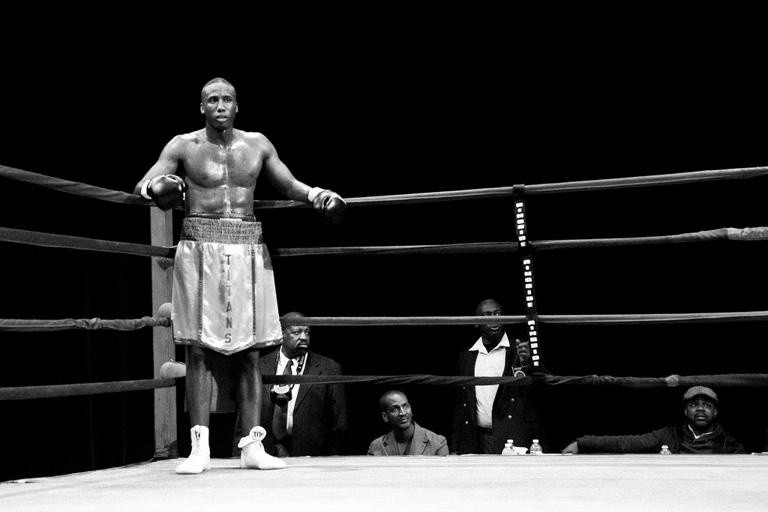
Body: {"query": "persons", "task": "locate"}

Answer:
[451,296,559,454]
[232,311,348,457]
[561,385,748,454]
[132,75,348,476]
[365,388,452,456]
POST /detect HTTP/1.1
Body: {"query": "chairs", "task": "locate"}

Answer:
[237,421,289,472]
[175,424,213,474]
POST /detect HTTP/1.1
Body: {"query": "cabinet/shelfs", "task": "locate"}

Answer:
[531,439,541,453]
[503,439,515,454]
[658,443,671,456]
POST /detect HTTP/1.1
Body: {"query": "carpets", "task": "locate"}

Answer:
[270,359,294,441]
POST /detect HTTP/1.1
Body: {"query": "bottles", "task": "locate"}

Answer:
[683,384,719,406]
